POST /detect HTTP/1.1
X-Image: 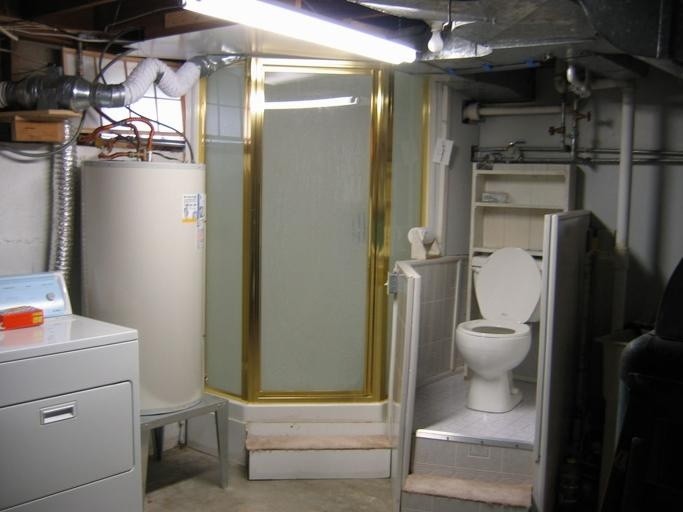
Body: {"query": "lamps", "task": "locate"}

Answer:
[426,23,445,53]
[263,95,358,111]
[180,0,422,66]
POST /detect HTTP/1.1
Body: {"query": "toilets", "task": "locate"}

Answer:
[455,246,543,414]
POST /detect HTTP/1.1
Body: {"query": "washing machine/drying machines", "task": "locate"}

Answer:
[0,271,144,507]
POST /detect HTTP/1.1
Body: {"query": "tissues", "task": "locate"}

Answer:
[409,227,433,249]
